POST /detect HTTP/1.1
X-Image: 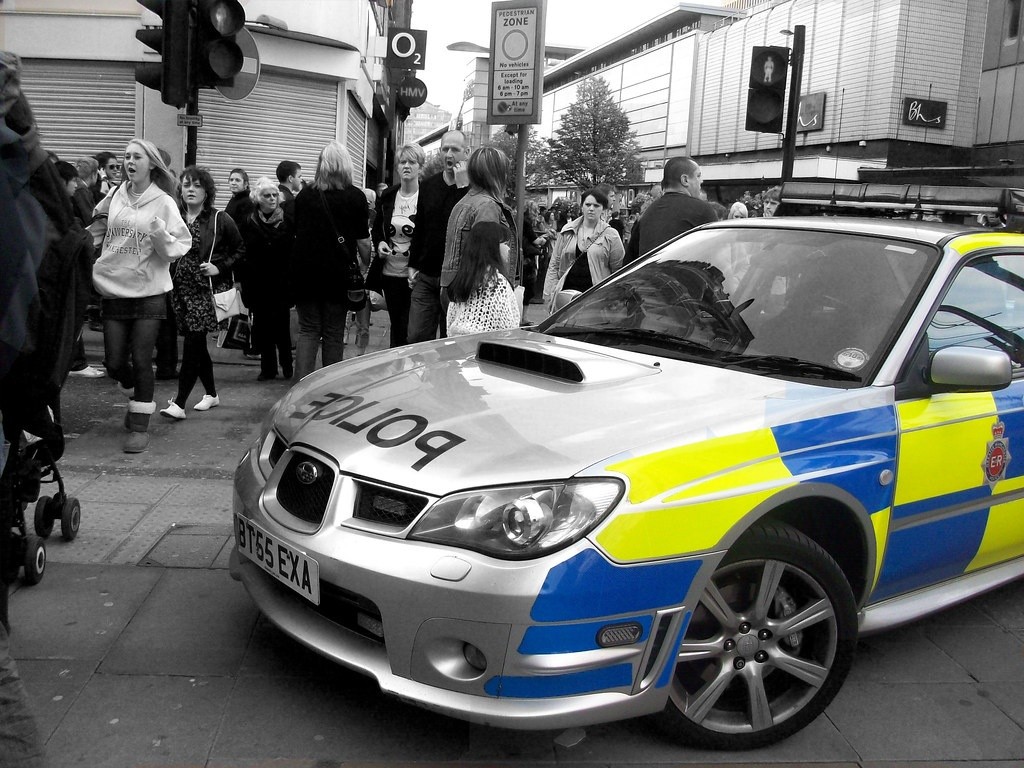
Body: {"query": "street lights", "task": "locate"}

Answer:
[445,40,531,292]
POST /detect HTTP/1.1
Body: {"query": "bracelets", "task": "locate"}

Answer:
[458,184,468,188]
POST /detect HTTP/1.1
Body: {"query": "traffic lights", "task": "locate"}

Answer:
[131,0,188,109]
[186,0,247,90]
[745,46,790,134]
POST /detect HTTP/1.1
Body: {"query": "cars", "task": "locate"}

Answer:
[225,180,1023,752]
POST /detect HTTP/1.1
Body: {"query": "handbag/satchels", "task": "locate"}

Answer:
[211,287,239,322]
[366,274,387,309]
[216,290,252,351]
[548,276,566,315]
[338,249,365,292]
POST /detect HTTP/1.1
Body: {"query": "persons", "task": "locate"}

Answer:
[515,183,640,327]
[42,136,253,453]
[405,129,472,345]
[623,157,783,268]
[372,142,424,348]
[0,50,65,639]
[438,146,518,340]
[243,141,388,386]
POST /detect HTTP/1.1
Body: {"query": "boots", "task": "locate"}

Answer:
[117,381,137,430]
[85,304,103,332]
[124,400,156,452]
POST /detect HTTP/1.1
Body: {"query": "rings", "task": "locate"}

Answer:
[457,165,460,167]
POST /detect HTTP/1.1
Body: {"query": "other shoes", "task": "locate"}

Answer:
[155,367,179,381]
[520,320,534,326]
[282,363,294,380]
[257,368,278,382]
[245,350,263,360]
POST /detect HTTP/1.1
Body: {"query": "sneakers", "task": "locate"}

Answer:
[193,393,220,410]
[67,366,104,378]
[159,397,187,420]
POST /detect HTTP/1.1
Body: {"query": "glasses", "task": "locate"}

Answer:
[262,193,279,198]
[107,164,120,170]
